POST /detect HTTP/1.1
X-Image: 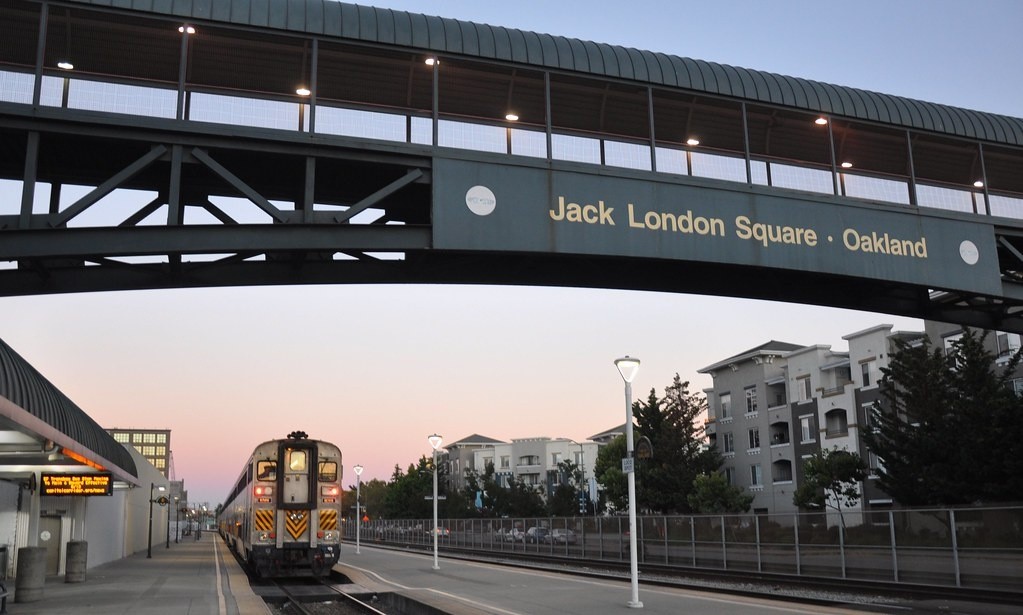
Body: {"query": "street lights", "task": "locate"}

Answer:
[613,354,646,609]
[55,61,73,110]
[839,162,852,196]
[424,56,443,148]
[175,21,195,120]
[147,483,166,558]
[426,434,444,572]
[351,463,363,556]
[166,493,179,548]
[815,113,839,195]
[686,138,703,179]
[970,180,985,215]
[504,113,519,154]
[296,86,311,132]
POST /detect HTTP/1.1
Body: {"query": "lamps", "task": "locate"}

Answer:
[687,134,700,146]
[296,81,312,96]
[178,23,200,37]
[814,115,827,126]
[155,485,165,492]
[421,54,441,67]
[503,109,520,123]
[841,158,854,168]
[48,447,65,461]
[56,55,74,71]
[111,479,136,489]
[973,176,984,187]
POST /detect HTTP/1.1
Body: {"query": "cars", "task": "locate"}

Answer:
[361,523,450,538]
[492,526,577,544]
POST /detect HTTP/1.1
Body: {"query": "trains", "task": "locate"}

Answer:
[214,431,343,582]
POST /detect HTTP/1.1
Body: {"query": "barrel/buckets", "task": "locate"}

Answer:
[14,547,47,604]
[65,540,88,583]
